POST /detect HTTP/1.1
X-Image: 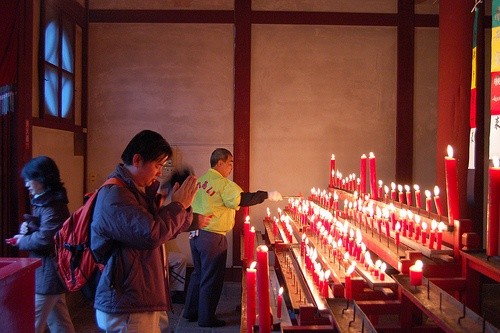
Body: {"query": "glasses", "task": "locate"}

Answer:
[154,159,167,172]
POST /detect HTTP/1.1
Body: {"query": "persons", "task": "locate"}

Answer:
[94,129,199,333]
[12,155,75,333]
[182,149,283,327]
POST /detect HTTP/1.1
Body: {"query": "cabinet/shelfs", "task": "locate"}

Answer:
[264,186,500,333]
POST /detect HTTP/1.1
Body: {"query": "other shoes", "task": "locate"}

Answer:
[183,315,198,322]
[198,318,225,328]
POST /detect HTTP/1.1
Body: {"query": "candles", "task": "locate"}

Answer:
[244,144,500,333]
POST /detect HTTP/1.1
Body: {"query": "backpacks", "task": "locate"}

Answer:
[53,178,135,295]
